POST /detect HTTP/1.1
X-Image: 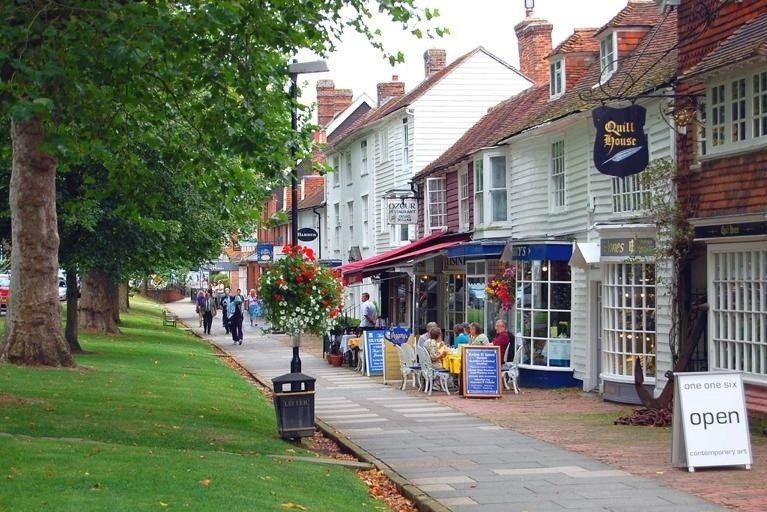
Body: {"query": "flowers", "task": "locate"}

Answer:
[256,244,343,337]
[487,264,517,311]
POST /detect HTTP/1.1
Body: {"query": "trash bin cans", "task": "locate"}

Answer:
[271,372,317,439]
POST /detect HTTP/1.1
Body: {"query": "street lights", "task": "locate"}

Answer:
[287,60,329,254]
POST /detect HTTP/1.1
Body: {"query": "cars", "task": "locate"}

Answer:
[57,268,81,301]
[0,273,11,312]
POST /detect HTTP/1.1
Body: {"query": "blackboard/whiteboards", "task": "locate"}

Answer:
[463,346,502,398]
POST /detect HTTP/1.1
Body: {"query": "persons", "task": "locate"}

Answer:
[416,317,510,394]
[359,292,378,331]
[171,274,264,346]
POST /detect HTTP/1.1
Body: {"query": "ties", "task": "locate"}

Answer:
[206,299,209,312]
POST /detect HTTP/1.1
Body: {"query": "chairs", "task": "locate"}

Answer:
[390,342,525,394]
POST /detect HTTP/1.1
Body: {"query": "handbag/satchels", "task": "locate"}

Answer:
[211,304,217,316]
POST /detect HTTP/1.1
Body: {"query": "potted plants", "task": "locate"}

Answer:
[326,326,345,367]
